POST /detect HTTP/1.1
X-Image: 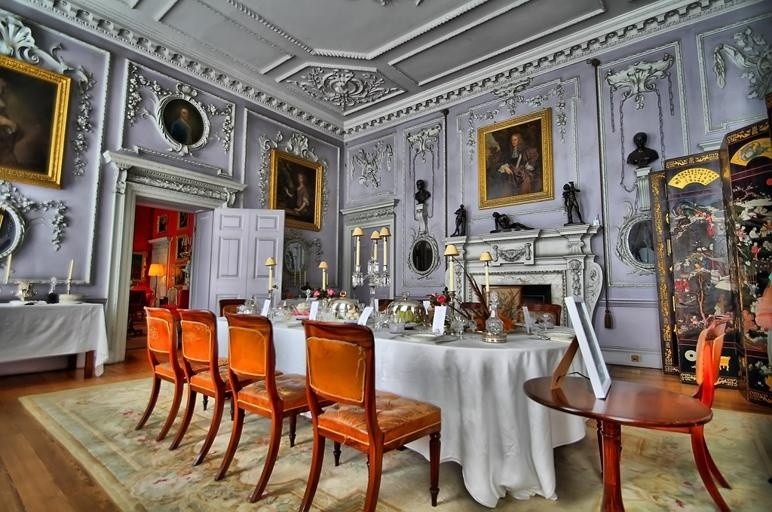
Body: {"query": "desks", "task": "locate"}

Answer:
[216,315,582,503]
[0,302,104,378]
[523,376,713,512]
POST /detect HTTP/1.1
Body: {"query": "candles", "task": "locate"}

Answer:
[5,254,12,284]
[69,259,74,279]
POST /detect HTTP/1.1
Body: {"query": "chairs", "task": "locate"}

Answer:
[170,307,296,466]
[127,291,149,341]
[596,316,733,512]
[135,305,228,451]
[212,313,343,503]
[299,318,442,512]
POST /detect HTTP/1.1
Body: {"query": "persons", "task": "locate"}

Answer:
[414,180,431,204]
[282,169,313,219]
[450,203,467,237]
[492,210,535,232]
[626,131,660,168]
[170,106,195,146]
[497,131,539,196]
[561,180,587,225]
[753,267,771,487]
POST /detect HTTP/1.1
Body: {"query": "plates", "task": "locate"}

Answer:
[405,331,439,341]
[539,330,574,341]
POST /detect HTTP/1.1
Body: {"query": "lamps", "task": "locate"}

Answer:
[480,252,493,292]
[148,263,165,307]
[319,261,329,291]
[443,245,459,291]
[264,257,277,290]
[352,226,391,311]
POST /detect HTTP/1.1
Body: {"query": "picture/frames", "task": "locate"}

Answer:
[0,52,73,190]
[477,106,555,210]
[268,148,324,232]
[157,216,167,232]
[550,296,612,400]
[173,212,191,286]
[131,251,147,282]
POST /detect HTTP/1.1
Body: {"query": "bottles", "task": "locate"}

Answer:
[484,290,505,336]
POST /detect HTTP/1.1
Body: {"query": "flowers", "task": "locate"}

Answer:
[313,288,336,303]
[425,287,458,322]
